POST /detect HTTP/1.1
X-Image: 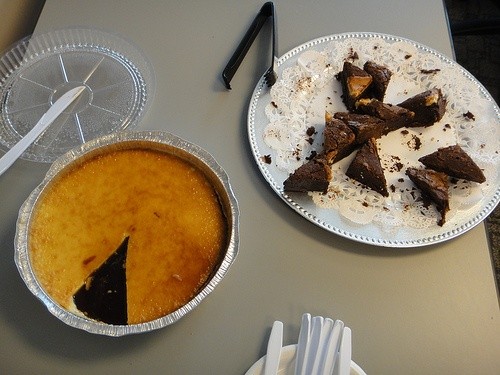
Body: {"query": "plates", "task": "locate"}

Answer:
[247,31,500,249]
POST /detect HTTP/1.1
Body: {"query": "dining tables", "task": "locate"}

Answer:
[0,0,499,375]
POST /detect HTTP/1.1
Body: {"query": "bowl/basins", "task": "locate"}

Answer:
[14,130,240,338]
[245,343,370,375]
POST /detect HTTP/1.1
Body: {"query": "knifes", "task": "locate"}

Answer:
[1,85,86,178]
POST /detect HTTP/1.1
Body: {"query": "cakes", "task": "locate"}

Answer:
[283,60,488,213]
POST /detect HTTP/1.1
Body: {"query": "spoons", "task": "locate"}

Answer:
[265,312,352,375]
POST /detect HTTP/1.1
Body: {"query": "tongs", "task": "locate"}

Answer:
[222,1,277,90]
